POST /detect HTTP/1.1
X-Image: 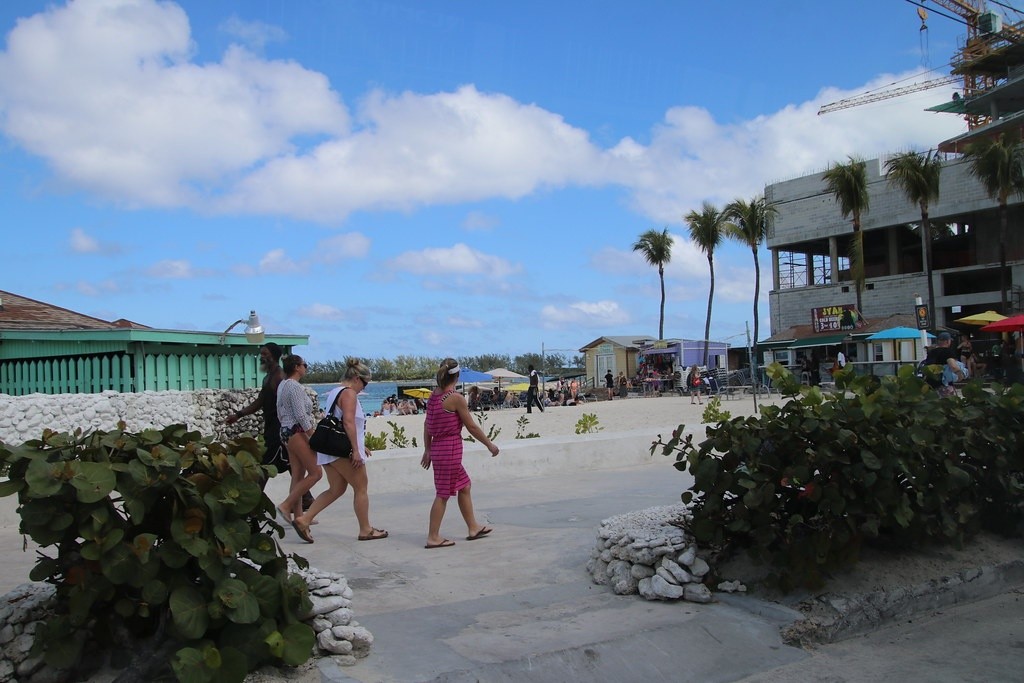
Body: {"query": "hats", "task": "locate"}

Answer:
[937,332,954,341]
[448,363,462,374]
[264,342,282,362]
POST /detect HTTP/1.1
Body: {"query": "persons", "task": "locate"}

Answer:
[703,372,713,394]
[223,343,315,511]
[292,356,388,543]
[526,365,543,413]
[381,394,426,415]
[801,348,845,388]
[468,386,509,411]
[421,358,499,549]
[604,370,628,400]
[653,368,659,391]
[540,378,580,406]
[275,356,323,525]
[927,332,1010,395]
[690,363,703,405]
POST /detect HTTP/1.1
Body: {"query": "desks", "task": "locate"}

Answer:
[724,385,753,401]
[757,365,802,399]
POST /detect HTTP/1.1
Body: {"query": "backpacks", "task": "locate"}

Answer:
[687,374,691,388]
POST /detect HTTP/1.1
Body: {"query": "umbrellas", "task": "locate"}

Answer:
[485,368,527,390]
[546,372,587,382]
[403,388,433,400]
[457,368,497,392]
[953,310,1024,358]
[865,325,938,359]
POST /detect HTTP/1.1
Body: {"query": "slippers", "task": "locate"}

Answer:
[276,507,292,525]
[292,522,314,543]
[309,519,318,525]
[425,539,455,548]
[467,527,493,540]
[358,527,388,540]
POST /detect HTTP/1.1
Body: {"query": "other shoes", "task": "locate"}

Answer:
[691,402,695,405]
[699,402,703,404]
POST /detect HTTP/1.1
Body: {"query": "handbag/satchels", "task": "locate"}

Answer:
[309,387,354,459]
[623,381,626,385]
[915,360,928,381]
[693,378,701,387]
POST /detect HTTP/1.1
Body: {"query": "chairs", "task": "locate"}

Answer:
[470,390,515,411]
[709,378,728,401]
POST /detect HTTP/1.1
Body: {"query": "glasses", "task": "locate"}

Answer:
[300,364,307,368]
[360,377,368,387]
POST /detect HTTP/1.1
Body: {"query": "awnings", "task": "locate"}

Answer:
[787,334,846,349]
